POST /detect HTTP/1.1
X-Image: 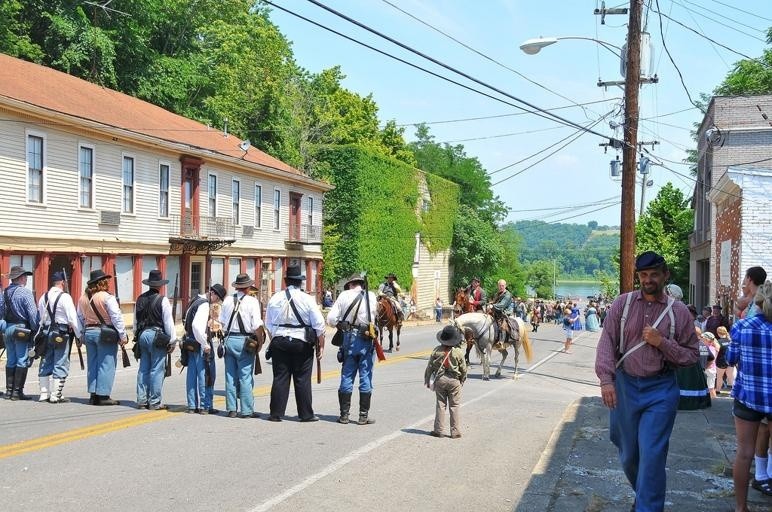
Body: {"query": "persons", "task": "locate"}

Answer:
[75,268,128,406]
[435,299,444,321]
[264,264,326,422]
[400,297,416,319]
[218,275,266,417]
[33,271,83,403]
[469,277,514,346]
[508,294,611,331]
[726,266,771,512]
[562,308,579,354]
[327,272,378,424]
[178,284,226,416]
[687,304,734,399]
[664,285,712,411]
[0,265,40,401]
[133,269,178,409]
[324,286,332,307]
[378,273,403,325]
[595,253,700,512]
[424,325,467,438]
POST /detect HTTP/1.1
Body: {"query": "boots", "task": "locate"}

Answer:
[38,375,51,403]
[357,392,376,426]
[4,366,32,401]
[49,377,71,403]
[336,389,352,425]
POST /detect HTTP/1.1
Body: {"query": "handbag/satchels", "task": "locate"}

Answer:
[242,337,260,356]
[217,341,227,360]
[304,325,318,345]
[132,339,142,361]
[51,329,70,346]
[153,331,171,349]
[331,330,344,347]
[359,324,377,341]
[100,326,119,344]
[13,326,33,343]
[34,329,50,359]
[429,374,437,392]
[183,338,201,354]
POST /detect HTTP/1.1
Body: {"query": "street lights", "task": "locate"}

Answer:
[520,35,642,295]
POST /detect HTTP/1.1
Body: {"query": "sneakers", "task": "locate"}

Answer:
[451,434,461,439]
[265,415,284,422]
[89,395,120,406]
[188,408,219,415]
[226,411,262,419]
[430,431,444,438]
[299,416,321,423]
[667,283,683,301]
[137,402,169,410]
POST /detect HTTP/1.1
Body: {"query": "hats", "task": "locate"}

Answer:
[384,272,398,282]
[344,273,365,290]
[283,265,306,280]
[86,269,113,286]
[231,274,256,291]
[207,283,227,303]
[436,325,462,347]
[142,270,170,288]
[51,271,67,283]
[635,251,665,272]
[0,266,33,281]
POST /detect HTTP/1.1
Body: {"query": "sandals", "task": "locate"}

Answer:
[752,476,771,496]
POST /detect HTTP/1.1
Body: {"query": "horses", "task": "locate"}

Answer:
[376,294,404,354]
[453,285,487,365]
[448,311,533,381]
